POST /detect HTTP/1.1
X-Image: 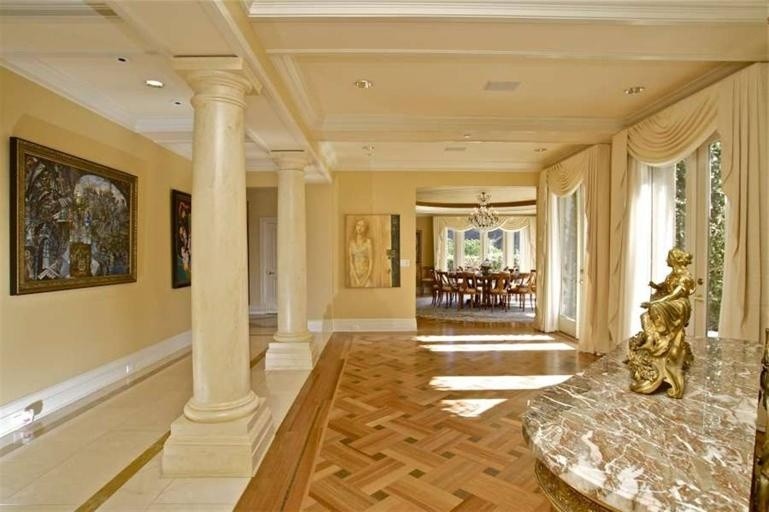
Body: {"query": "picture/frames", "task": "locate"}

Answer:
[170,189,192,290]
[9,136,137,294]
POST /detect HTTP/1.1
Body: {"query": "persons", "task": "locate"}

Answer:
[632,244,694,353]
[348,216,375,286]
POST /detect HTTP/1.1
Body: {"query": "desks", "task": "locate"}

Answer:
[523,334,765,511]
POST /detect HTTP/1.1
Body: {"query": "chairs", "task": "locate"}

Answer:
[420,268,536,314]
